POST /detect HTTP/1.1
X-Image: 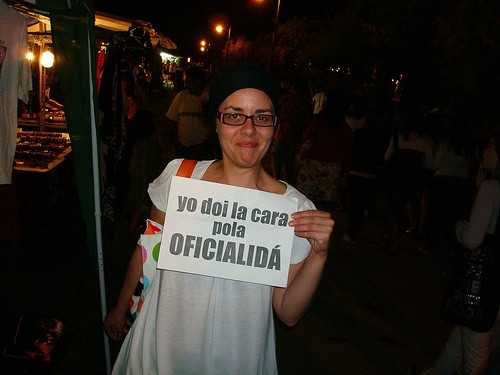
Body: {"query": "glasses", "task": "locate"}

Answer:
[215,110,277,128]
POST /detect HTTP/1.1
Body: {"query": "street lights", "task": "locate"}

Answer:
[216,24,231,67]
[200,40,211,65]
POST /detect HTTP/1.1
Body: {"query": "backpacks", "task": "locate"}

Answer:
[127,158,198,316]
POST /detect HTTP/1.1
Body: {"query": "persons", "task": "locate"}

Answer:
[128,66,500,310]
[409,129,500,375]
[103,77,335,375]
